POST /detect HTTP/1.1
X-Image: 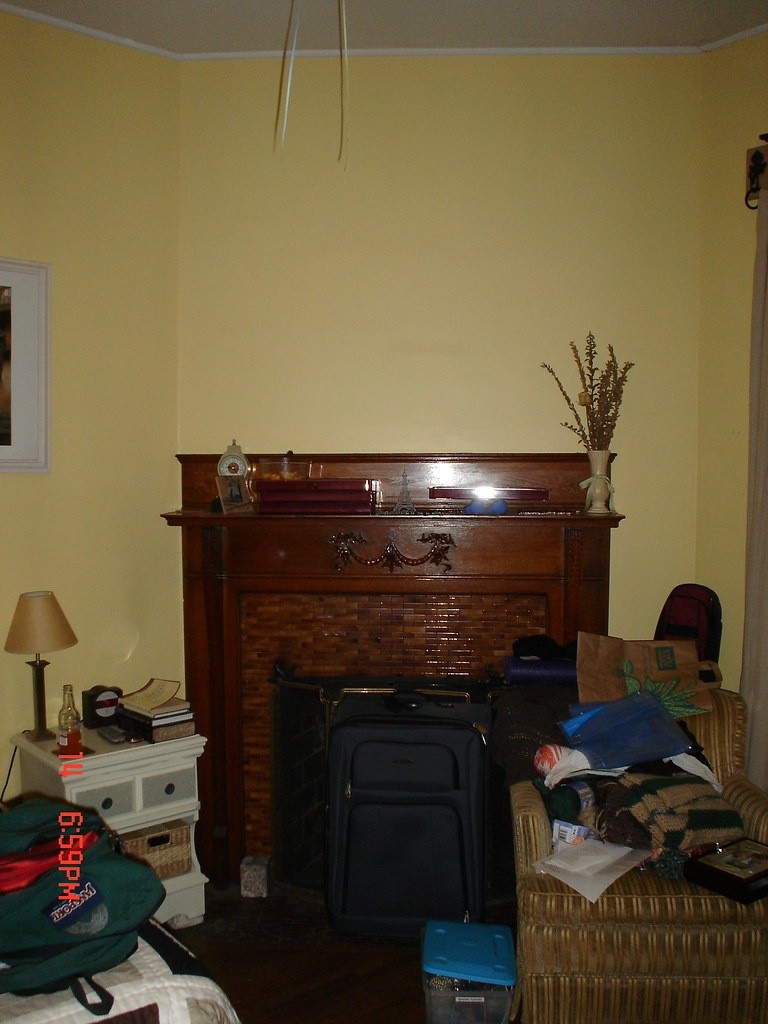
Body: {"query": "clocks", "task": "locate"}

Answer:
[217,439,251,479]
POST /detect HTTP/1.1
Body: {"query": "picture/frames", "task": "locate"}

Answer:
[215,475,249,513]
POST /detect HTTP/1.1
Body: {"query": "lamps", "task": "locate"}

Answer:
[4,590,79,742]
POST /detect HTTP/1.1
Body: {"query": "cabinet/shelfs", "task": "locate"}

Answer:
[10,723,209,929]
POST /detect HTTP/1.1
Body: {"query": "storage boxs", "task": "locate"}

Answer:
[420,919,518,1024]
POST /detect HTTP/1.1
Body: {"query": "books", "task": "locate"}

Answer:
[115,678,196,744]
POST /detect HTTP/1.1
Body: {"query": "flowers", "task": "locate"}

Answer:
[539,329,635,450]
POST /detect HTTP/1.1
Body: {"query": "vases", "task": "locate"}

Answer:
[586,450,611,514]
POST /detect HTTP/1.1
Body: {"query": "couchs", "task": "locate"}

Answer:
[508,688,768,1024]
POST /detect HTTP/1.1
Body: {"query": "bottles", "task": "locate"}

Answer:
[57,685,83,755]
